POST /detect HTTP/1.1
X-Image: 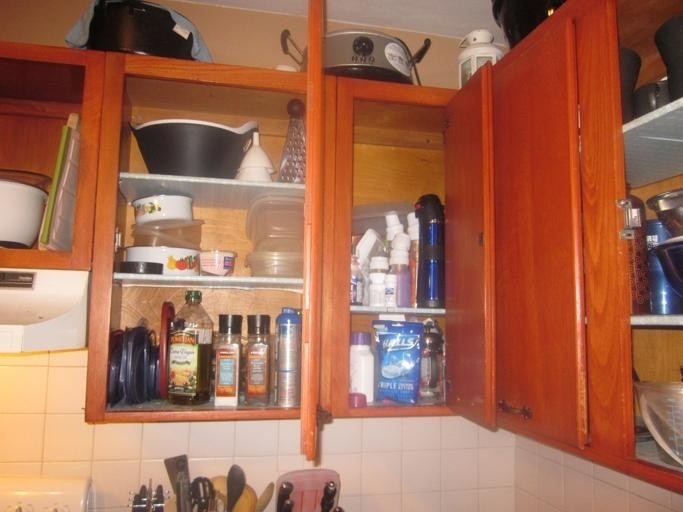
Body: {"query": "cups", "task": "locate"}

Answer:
[633,83,668,117]
[236,132,276,182]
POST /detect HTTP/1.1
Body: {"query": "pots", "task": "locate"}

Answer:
[86,4,162,57]
[279,27,432,86]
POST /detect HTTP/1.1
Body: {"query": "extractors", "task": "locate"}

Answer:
[0,267,83,357]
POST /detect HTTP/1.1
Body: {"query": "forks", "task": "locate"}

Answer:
[206,499,216,512]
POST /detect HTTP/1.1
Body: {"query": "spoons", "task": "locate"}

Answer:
[224,464,245,511]
[191,477,212,512]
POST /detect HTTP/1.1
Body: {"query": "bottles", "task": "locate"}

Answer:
[349,194,445,309]
[350,330,373,403]
[455,30,504,90]
[167,287,304,410]
[279,99,307,185]
[418,321,444,404]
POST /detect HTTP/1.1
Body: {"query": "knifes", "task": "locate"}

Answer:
[275,481,293,512]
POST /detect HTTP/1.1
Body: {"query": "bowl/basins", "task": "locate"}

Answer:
[127,117,256,179]
[0,179,48,250]
[644,187,683,298]
[114,193,238,278]
[634,377,683,468]
